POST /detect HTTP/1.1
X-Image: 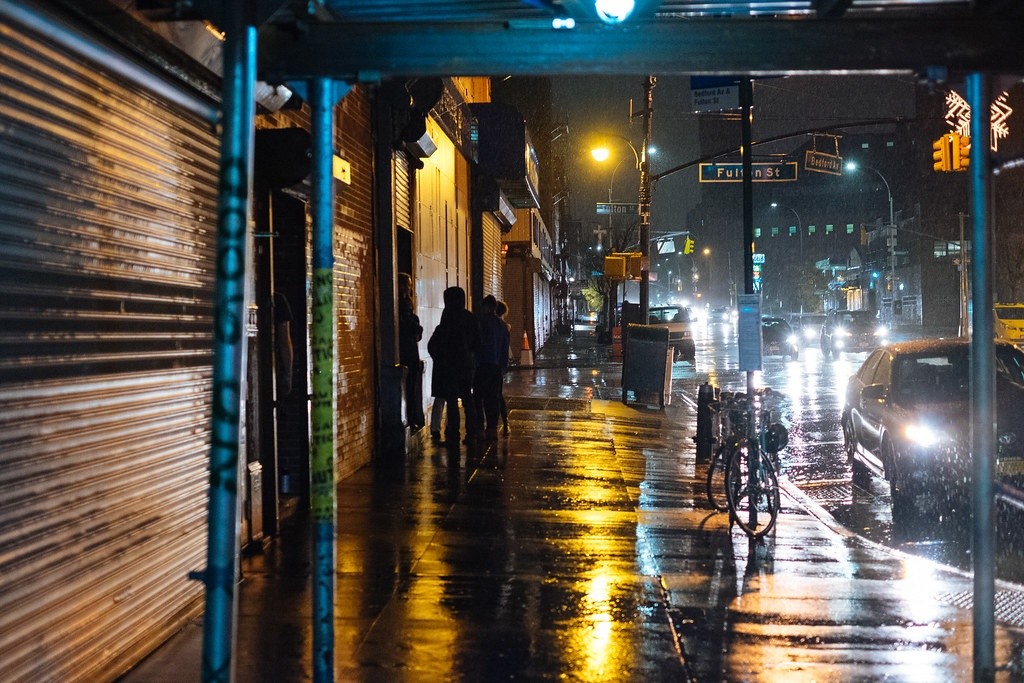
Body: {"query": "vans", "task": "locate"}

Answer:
[990,302,1024,347]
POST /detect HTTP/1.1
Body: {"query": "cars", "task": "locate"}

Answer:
[706,307,730,326]
[760,316,800,362]
[838,338,1023,521]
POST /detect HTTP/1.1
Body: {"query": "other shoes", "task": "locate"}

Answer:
[432,426,512,446]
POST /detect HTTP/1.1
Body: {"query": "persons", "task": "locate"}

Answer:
[427,286,511,450]
[398,272,422,433]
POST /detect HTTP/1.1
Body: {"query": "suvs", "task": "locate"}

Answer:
[648,302,701,359]
[821,313,889,357]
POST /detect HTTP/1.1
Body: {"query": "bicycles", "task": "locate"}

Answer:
[703,387,786,537]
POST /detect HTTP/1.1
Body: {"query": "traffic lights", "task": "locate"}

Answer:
[684,238,696,255]
[950,133,972,175]
[629,254,642,277]
[932,135,951,174]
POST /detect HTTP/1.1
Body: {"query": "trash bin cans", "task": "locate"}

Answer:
[634,347,674,405]
[620,322,670,410]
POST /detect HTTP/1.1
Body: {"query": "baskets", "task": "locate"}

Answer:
[707,401,748,441]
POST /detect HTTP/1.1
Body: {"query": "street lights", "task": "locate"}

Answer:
[771,203,804,265]
[589,132,656,321]
[606,146,658,251]
[845,162,902,314]
